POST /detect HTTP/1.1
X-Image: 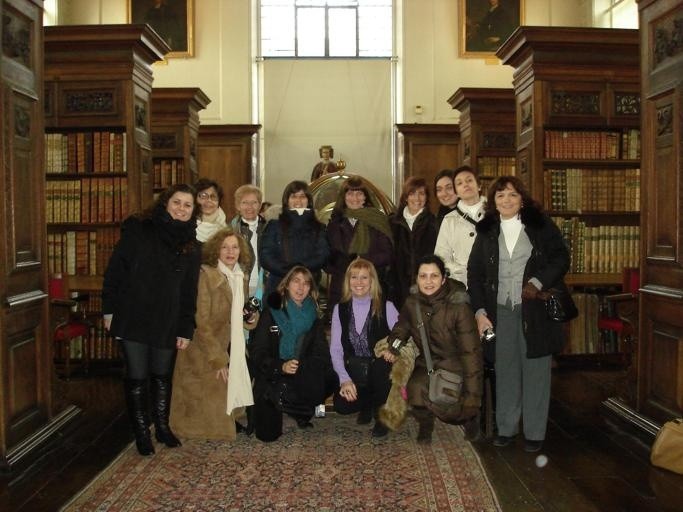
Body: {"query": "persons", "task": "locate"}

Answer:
[383,255,485,446]
[166,229,260,443]
[247,265,336,442]
[467,176,570,454]
[466,0,513,51]
[191,177,228,258]
[227,184,274,302]
[100,184,201,454]
[325,176,394,292]
[260,181,328,301]
[143,0,186,51]
[379,166,489,307]
[311,145,337,183]
[328,259,401,423]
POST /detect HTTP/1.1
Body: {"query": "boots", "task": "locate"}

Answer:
[128,377,156,456]
[154,374,182,448]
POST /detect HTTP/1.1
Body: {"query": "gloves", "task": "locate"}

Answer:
[521,282,537,300]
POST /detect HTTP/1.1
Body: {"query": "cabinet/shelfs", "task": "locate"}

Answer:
[198,124,261,219]
[636,0,683,446]
[395,123,460,213]
[495,24,642,373]
[1,0,57,470]
[447,86,517,197]
[44,24,172,407]
[152,86,212,200]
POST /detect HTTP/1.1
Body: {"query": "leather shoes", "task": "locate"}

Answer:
[295,416,315,430]
[492,432,515,447]
[523,438,545,454]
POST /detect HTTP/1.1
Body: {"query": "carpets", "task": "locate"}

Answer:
[59,397,503,511]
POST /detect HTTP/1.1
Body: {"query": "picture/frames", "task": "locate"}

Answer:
[459,1,526,58]
[123,1,194,59]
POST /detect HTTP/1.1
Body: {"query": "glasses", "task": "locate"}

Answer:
[197,191,221,202]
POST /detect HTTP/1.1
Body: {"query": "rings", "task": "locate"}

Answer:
[347,392,350,394]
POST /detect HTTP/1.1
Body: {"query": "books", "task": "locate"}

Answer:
[44,132,127,361]
[477,156,516,196]
[544,128,642,356]
[153,158,182,188]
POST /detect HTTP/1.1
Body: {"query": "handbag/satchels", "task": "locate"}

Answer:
[346,355,375,389]
[250,374,292,412]
[427,369,463,405]
[543,288,580,324]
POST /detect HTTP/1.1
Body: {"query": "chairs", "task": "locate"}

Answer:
[49,274,90,383]
[598,266,639,365]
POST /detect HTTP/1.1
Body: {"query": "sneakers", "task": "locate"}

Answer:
[462,413,483,443]
[357,411,374,425]
[372,420,392,444]
[415,414,434,445]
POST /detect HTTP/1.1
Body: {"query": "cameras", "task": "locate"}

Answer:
[243,297,261,320]
[481,327,495,341]
